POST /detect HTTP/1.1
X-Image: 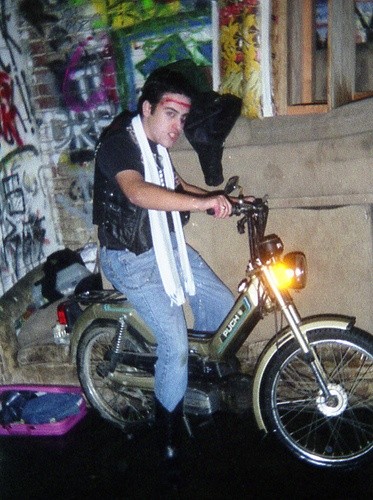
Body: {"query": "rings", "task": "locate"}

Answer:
[222,206,227,211]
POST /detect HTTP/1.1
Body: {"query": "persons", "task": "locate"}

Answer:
[92,73,254,457]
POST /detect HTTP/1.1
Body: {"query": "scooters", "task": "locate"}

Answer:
[56,174,373,473]
